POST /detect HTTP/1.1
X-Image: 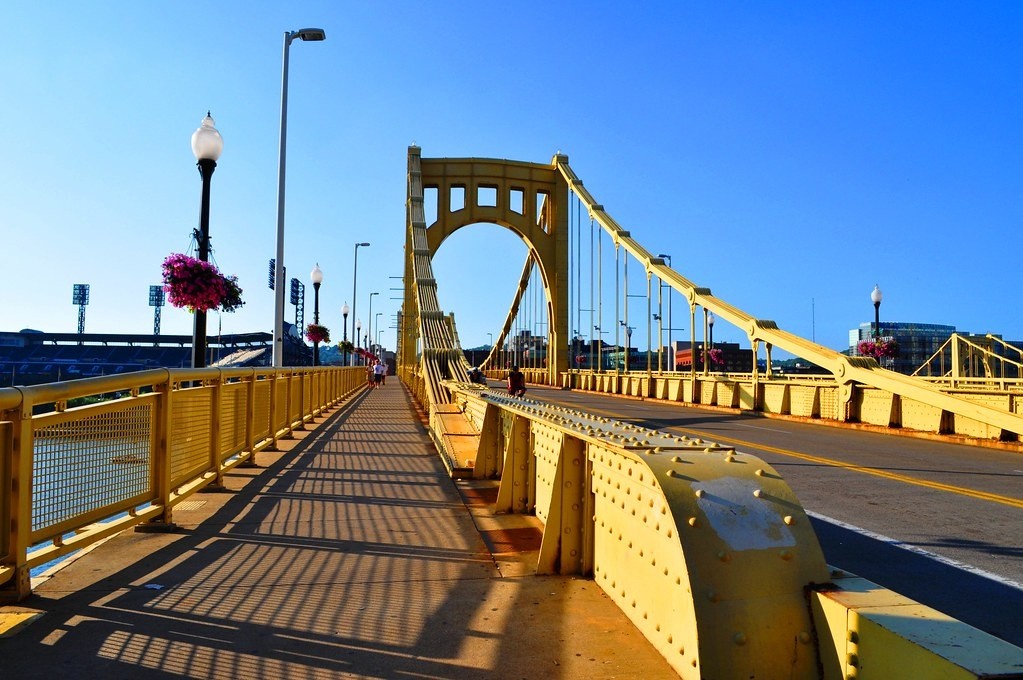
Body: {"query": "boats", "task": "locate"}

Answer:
[111,454,144,464]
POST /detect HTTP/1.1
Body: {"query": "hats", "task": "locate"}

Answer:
[473,367,478,372]
[513,366,519,371]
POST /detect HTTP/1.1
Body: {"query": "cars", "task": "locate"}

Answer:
[94,391,122,400]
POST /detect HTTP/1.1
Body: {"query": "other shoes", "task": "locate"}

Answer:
[375,386,377,389]
[371,386,373,390]
[377,386,380,390]
[368,386,370,389]
[383,383,385,385]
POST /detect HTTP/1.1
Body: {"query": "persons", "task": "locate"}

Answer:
[467,367,487,386]
[499,366,527,397]
[365,359,388,389]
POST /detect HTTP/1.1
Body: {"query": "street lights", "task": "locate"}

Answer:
[627,326,632,368]
[352,243,370,365]
[708,313,714,374]
[273,26,326,367]
[364,330,368,365]
[342,302,350,367]
[369,292,379,365]
[192,111,222,390]
[872,285,883,359]
[657,253,672,372]
[374,312,382,355]
[356,318,362,366]
[310,262,326,365]
[486,332,492,350]
[379,330,384,358]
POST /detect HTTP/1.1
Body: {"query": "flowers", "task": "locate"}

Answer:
[169,253,246,316]
[575,353,588,364]
[698,346,726,368]
[304,321,382,366]
[863,334,899,360]
